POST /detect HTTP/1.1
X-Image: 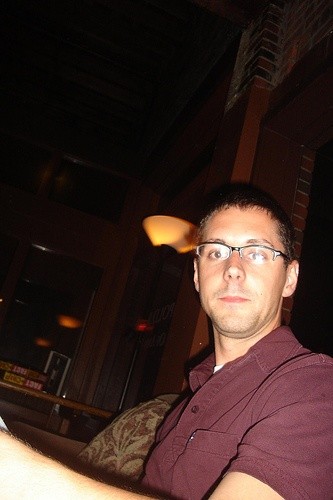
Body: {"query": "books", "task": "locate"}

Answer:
[0,360,48,384]
[3,372,45,392]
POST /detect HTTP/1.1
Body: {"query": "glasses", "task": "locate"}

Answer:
[196,242,289,262]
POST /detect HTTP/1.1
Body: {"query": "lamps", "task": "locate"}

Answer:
[142,214,201,257]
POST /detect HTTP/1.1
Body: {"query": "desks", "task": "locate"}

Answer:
[0,379,116,436]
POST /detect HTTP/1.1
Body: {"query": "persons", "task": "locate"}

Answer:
[0,190,333,500]
